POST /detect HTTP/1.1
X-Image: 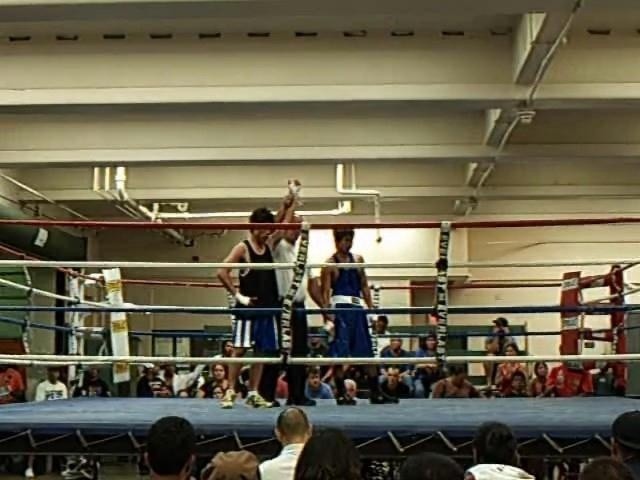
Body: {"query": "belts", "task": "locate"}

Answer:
[331,295,363,306]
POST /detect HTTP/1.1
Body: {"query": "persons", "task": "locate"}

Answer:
[1,316,639,478]
[321,226,401,405]
[258,194,333,406]
[216,178,303,410]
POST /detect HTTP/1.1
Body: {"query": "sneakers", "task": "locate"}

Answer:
[337,394,355,406]
[220,391,236,408]
[24,467,35,480]
[246,393,280,409]
[286,395,315,406]
[370,394,399,404]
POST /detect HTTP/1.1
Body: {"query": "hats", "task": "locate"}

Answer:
[492,317,508,327]
